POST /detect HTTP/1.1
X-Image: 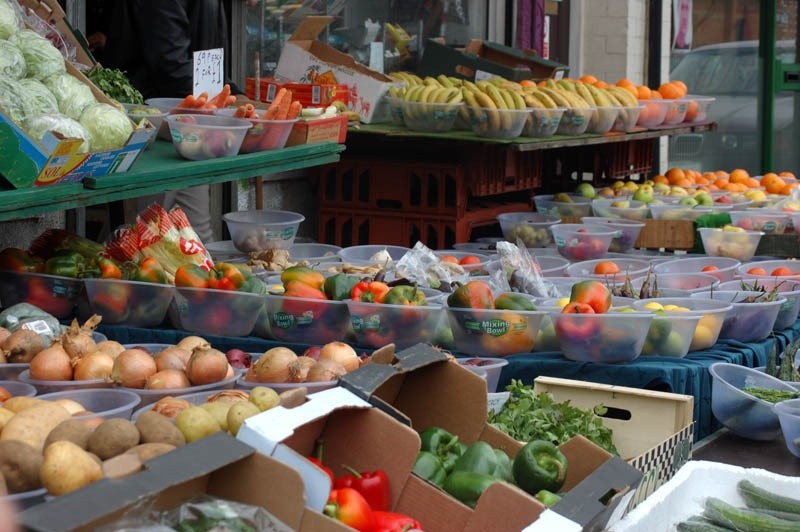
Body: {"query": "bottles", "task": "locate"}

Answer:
[247,28,287,78]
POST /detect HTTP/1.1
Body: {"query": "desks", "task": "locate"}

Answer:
[0,136,346,239]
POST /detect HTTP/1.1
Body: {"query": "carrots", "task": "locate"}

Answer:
[166,84,302,152]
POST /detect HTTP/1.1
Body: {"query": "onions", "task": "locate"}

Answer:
[0,316,372,384]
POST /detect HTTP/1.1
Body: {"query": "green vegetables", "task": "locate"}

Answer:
[84,64,144,106]
[486,378,622,459]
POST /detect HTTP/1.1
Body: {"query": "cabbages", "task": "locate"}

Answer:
[0,0,134,156]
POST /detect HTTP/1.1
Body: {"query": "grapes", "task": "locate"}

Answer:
[506,222,549,248]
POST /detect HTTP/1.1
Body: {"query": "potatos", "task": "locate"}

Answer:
[0,386,308,502]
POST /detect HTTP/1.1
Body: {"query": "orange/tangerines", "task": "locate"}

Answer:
[653,167,800,197]
[523,75,704,127]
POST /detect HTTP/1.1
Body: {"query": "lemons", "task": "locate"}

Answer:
[533,299,721,358]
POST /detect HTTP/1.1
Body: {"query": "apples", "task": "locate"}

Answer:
[574,179,766,208]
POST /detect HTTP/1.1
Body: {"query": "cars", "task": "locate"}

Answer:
[669,38,800,180]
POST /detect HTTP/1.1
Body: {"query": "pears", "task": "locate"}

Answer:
[707,224,755,259]
[546,194,800,235]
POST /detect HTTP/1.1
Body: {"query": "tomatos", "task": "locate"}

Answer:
[594,261,620,273]
[694,265,799,290]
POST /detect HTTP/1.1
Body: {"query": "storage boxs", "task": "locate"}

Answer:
[316,138,656,251]
[234,16,570,146]
[0,1,155,189]
[14,344,800,532]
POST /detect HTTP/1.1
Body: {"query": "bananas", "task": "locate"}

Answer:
[382,70,640,137]
[330,101,362,121]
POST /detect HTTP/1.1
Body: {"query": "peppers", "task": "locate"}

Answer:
[172,428,572,532]
[0,247,268,336]
[265,265,640,363]
[605,262,663,298]
[721,278,799,344]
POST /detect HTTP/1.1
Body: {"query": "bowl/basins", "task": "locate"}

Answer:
[581,217,646,253]
[712,176,800,235]
[556,109,597,136]
[550,224,618,261]
[0,331,339,514]
[697,227,765,260]
[204,210,492,292]
[444,238,734,362]
[692,256,800,341]
[664,95,716,124]
[145,98,219,143]
[456,358,509,393]
[164,109,300,160]
[639,99,671,127]
[465,107,533,140]
[585,109,620,134]
[612,107,644,132]
[14,271,444,354]
[497,212,562,249]
[521,108,568,138]
[385,95,465,133]
[708,361,800,458]
[123,103,170,150]
[533,185,734,227]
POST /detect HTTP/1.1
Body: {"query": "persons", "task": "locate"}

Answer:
[86,0,249,101]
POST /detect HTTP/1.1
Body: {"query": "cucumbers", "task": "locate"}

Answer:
[674,478,800,532]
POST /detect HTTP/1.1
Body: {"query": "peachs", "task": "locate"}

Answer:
[557,228,605,258]
